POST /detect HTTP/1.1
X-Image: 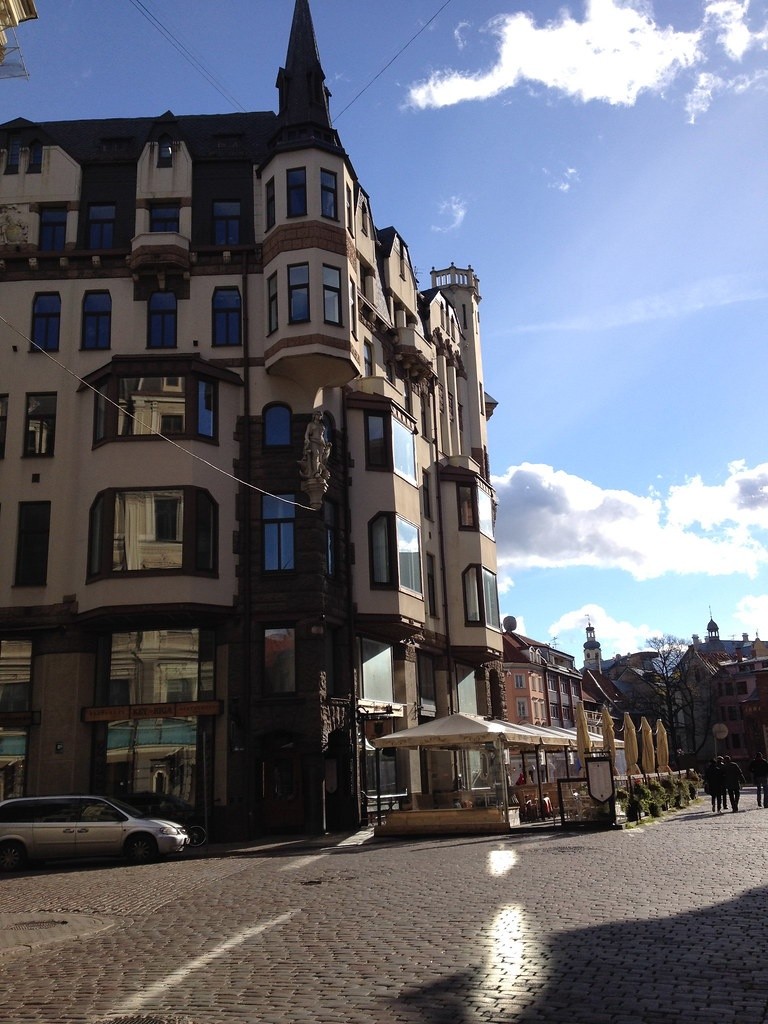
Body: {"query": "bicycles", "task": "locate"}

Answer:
[186,825,208,847]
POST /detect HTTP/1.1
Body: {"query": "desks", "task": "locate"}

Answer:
[563,797,598,822]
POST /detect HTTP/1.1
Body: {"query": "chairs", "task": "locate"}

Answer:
[549,790,576,826]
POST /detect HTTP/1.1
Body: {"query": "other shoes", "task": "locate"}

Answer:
[724,807,728,809]
[764,805,768,808]
[758,803,761,807]
[732,804,738,813]
[717,805,721,811]
[712,804,715,812]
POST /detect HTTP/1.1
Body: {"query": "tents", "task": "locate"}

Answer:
[371,712,625,826]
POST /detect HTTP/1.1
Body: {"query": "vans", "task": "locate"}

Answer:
[0,794,192,873]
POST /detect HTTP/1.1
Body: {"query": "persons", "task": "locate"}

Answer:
[516,773,526,785]
[453,774,465,790]
[752,752,768,808]
[545,758,556,783]
[303,410,332,475]
[703,756,746,813]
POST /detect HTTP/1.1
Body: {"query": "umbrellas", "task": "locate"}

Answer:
[624,713,641,775]
[576,701,593,777]
[641,717,655,774]
[656,719,673,773]
[602,707,620,776]
